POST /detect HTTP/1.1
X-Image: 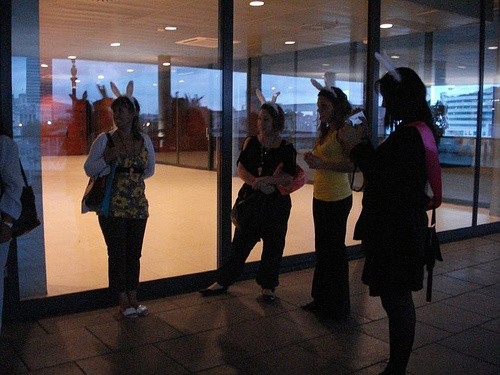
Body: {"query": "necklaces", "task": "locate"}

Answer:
[118,132,128,151]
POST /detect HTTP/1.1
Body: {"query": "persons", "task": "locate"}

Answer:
[200,103,297,301]
[0,134,24,331]
[82,95,156,318]
[339,67,440,375]
[302,86,360,319]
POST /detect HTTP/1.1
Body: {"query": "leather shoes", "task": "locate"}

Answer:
[261,289,275,302]
[200,286,228,296]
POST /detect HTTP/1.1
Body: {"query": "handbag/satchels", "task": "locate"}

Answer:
[83,132,114,207]
[9,157,41,238]
[423,226,443,272]
[230,183,263,236]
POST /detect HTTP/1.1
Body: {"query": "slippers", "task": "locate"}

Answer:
[121,304,150,318]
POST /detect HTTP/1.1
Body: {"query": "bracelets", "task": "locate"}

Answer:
[4,221,13,228]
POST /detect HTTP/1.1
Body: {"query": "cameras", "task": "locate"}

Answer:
[347,109,367,127]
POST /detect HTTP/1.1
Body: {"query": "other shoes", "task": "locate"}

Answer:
[300,300,326,311]
